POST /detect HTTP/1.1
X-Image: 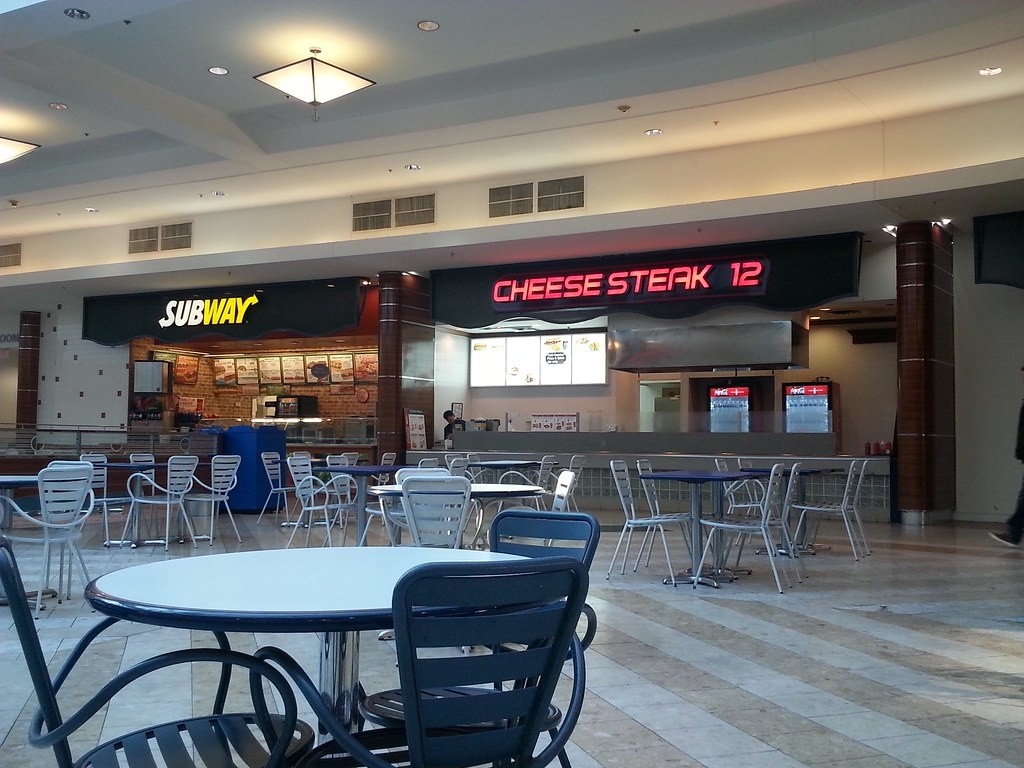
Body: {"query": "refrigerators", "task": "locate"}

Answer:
[706,381,764,433]
[782,380,842,454]
[275,394,318,418]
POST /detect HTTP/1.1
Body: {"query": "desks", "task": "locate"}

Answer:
[91,462,225,548]
[0,474,61,610]
[466,460,559,514]
[637,471,788,589]
[739,466,844,559]
[83,545,565,768]
[309,464,448,546]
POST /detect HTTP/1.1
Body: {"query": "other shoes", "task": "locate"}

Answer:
[989,531,1020,547]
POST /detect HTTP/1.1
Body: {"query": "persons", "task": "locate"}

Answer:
[988,366,1024,548]
[442,410,466,441]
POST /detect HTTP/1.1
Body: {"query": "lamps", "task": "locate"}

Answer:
[251,47,377,125]
[0,136,42,166]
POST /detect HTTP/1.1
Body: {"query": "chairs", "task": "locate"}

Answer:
[4,451,872,610]
[0,543,314,768]
[248,556,590,768]
[356,509,601,768]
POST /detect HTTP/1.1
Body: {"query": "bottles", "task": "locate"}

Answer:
[279,401,297,413]
[886,440,891,454]
[873,440,879,454]
[880,439,885,454]
[787,399,827,412]
[710,396,748,414]
[865,440,872,457]
